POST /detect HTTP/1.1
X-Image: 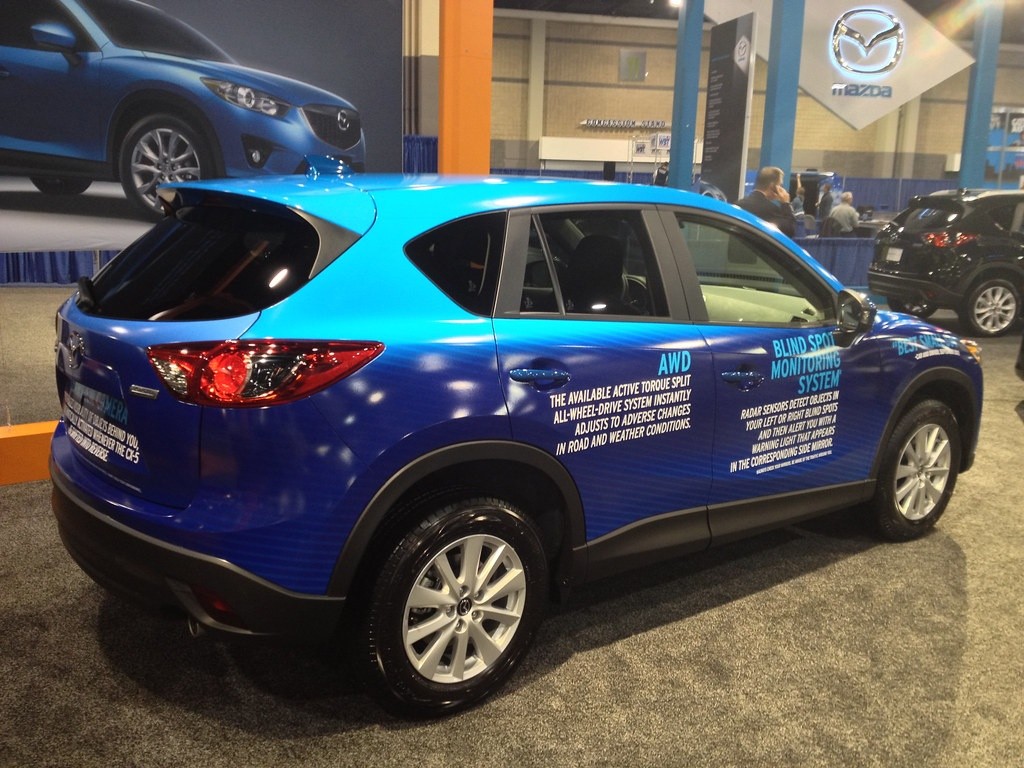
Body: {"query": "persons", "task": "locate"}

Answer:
[792,184,860,238]
[727,166,796,293]
[653,161,669,186]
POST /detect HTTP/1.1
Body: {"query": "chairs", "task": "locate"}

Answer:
[820,217,843,237]
[547,236,641,315]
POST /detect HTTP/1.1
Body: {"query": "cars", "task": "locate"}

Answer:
[42,170,987,715]
[0,1,365,230]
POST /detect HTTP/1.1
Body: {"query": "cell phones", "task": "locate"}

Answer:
[772,186,778,195]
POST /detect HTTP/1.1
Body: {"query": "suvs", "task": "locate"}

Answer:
[864,186,1024,339]
